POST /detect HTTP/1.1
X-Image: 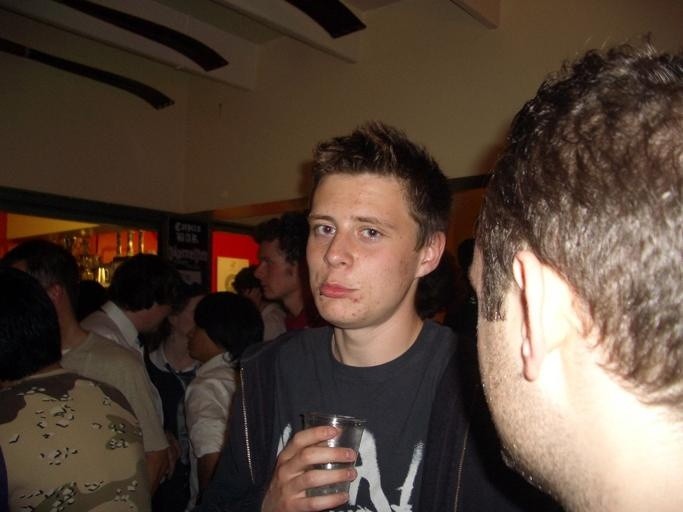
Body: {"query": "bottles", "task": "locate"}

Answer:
[66,226,142,288]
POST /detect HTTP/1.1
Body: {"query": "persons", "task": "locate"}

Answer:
[192,122,519,512]
[416,272,451,325]
[12,235,170,501]
[73,211,308,511]
[470,35,683,512]
[458,239,474,276]
[0,263,149,511]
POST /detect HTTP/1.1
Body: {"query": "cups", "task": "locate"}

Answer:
[304,410,366,501]
